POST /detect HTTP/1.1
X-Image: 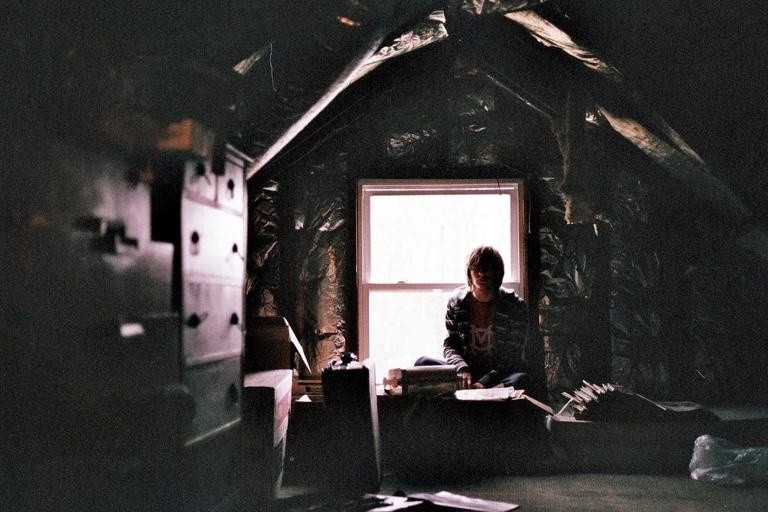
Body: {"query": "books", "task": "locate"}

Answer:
[559,378,673,423]
[397,381,459,396]
[399,364,457,383]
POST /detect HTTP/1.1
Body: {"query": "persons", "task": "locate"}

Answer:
[413,244,533,394]
